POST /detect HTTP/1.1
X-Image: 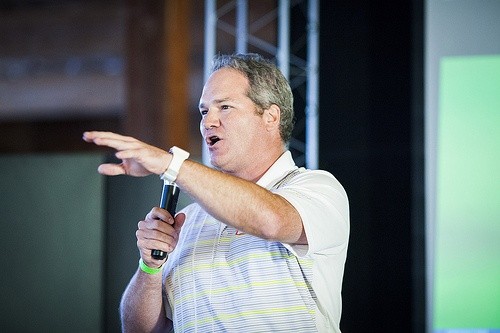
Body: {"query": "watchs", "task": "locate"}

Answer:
[160,145,191,183]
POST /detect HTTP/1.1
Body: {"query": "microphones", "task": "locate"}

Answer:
[152,181,181,259]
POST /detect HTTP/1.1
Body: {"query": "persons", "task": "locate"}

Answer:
[82,52,351,333]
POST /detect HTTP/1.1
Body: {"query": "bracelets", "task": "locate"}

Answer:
[138,258,162,275]
